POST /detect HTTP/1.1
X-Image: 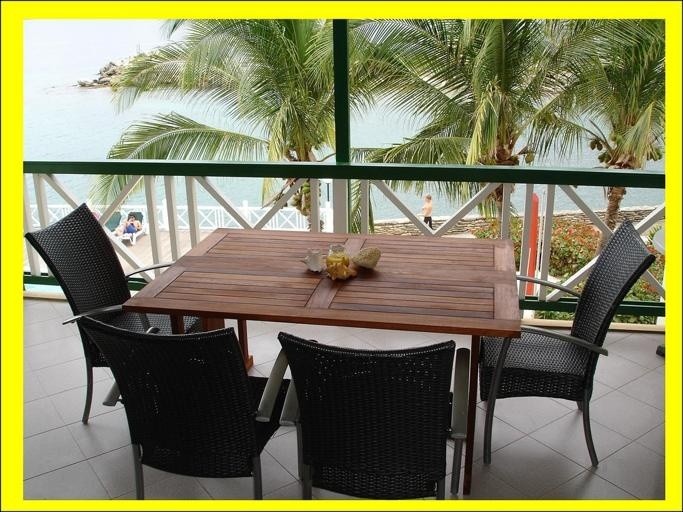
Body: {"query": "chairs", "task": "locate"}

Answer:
[474,215,655,466]
[275,330,472,500]
[25,200,203,425]
[79,315,288,500]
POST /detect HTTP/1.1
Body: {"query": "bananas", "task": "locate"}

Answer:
[327,253,356,279]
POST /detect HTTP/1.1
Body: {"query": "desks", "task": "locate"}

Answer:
[121,227,527,422]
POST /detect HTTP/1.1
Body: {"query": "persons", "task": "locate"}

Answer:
[114,213,142,236]
[421,194,432,229]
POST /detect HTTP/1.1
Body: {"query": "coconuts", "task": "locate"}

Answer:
[291,182,310,216]
[647,148,663,161]
